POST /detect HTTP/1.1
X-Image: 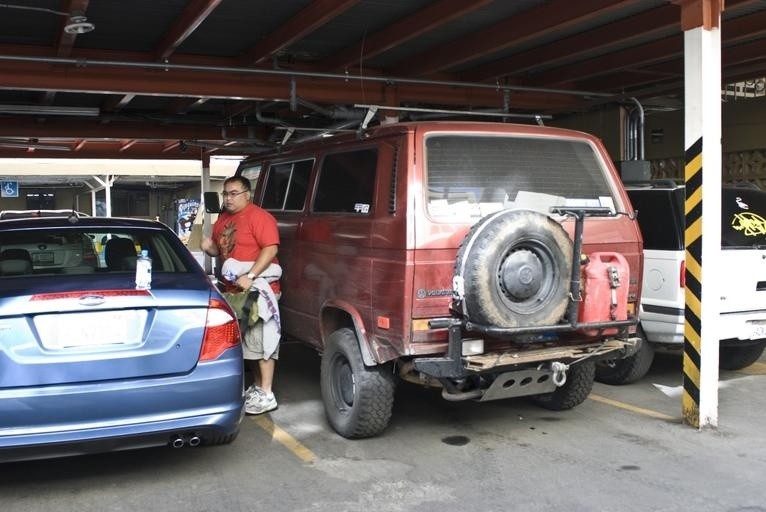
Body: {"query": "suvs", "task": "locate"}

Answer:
[595,177,766,387]
[202,118,645,441]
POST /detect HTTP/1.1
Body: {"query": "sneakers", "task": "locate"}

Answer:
[244,383,278,414]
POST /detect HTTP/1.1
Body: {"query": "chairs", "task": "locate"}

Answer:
[103,238,138,272]
[1,248,33,276]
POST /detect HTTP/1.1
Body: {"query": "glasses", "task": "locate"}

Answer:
[220,190,248,197]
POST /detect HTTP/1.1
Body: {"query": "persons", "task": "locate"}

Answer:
[198,175,283,416]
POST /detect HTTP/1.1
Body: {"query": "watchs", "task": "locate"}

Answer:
[246,272,256,281]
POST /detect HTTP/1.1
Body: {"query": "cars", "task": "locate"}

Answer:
[0,209,100,276]
[1,215,243,465]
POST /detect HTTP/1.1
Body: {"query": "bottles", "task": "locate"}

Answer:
[135,250,152,290]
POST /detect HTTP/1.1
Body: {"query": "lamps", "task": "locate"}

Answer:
[63,8,96,37]
